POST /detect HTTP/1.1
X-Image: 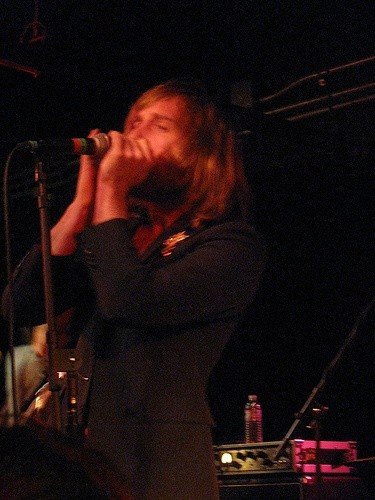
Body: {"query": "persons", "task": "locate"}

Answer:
[0,255,73,432]
[1,78,269,500]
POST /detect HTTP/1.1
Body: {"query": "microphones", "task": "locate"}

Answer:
[18,133,111,156]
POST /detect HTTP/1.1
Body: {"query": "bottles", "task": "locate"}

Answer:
[244,396,263,444]
